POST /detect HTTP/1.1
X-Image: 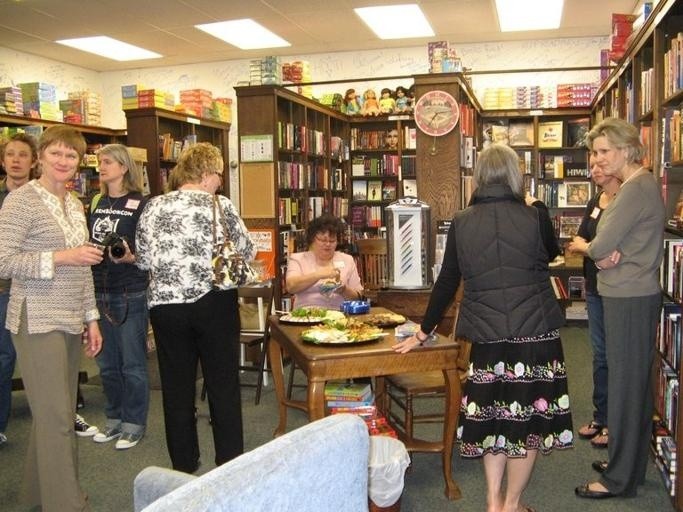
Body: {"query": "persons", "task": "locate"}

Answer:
[390,144,574,512]
[0,124,104,512]
[566,116,666,499]
[284,212,365,313]
[0,129,96,448]
[566,150,622,450]
[344,84,416,117]
[84,144,146,451]
[133,141,257,472]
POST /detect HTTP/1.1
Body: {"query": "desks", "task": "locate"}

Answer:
[265,305,463,502]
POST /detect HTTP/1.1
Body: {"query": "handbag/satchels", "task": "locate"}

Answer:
[212,193,258,291]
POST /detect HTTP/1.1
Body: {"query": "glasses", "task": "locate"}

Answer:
[315,234,338,247]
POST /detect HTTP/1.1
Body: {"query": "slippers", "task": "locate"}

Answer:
[577,420,605,437]
[591,428,608,448]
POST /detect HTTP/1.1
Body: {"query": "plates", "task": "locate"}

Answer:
[339,300,369,313]
[368,322,404,329]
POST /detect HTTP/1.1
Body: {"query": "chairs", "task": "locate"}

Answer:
[373,299,473,474]
[129,411,373,512]
[201,285,277,406]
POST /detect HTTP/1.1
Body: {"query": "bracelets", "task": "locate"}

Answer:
[415,333,428,346]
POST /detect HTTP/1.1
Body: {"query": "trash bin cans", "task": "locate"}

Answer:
[568,275,585,302]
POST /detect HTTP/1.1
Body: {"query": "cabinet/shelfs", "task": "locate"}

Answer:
[122,107,231,199]
[592,1,682,509]
[0,113,126,219]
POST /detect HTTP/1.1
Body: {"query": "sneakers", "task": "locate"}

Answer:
[92,426,122,443]
[0,433,7,446]
[115,431,143,449]
[74,413,99,437]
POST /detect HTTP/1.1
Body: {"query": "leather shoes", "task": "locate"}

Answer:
[575,483,617,498]
[592,460,608,473]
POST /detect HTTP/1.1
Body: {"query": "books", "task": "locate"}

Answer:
[482,83,595,149]
[428,41,473,87]
[277,122,348,191]
[517,151,591,237]
[279,194,349,257]
[1,81,105,198]
[236,57,345,113]
[120,84,235,187]
[458,103,478,209]
[352,125,400,238]
[595,0,683,494]
[548,256,568,298]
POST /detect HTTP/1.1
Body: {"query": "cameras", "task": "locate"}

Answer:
[101,232,133,257]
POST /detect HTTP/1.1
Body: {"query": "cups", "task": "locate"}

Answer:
[431,47,463,73]
[82,240,105,266]
